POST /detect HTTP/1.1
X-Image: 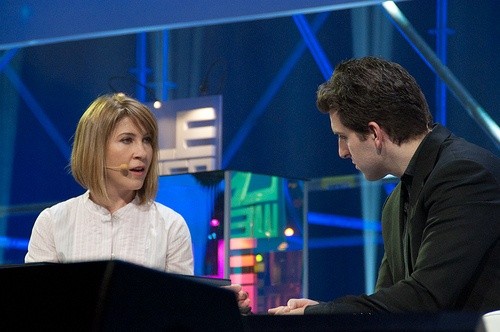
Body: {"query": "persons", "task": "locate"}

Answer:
[267,58,500,332]
[23,93,252,316]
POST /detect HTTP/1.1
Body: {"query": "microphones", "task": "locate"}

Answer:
[103,163,129,170]
